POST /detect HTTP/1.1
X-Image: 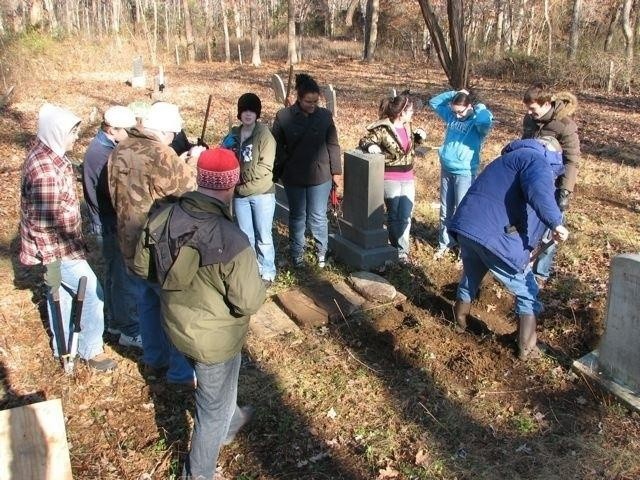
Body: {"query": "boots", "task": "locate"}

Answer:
[518,314,547,362]
[451,299,470,334]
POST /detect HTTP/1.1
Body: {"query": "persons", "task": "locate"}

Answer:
[271,74,343,270]
[445,133,569,362]
[518,83,580,291]
[429,88,494,270]
[82,100,215,388]
[131,148,268,480]
[16,101,118,372]
[359,94,427,267]
[219,93,276,289]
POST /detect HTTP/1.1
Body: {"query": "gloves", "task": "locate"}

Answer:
[556,188,569,212]
[44,261,61,295]
[222,133,239,148]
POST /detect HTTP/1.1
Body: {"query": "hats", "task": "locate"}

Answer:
[197,148,241,190]
[103,102,185,133]
[238,93,261,114]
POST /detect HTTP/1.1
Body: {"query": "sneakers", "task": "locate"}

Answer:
[261,255,326,289]
[85,327,143,372]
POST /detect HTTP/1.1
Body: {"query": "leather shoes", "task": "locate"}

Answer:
[238,404,256,433]
